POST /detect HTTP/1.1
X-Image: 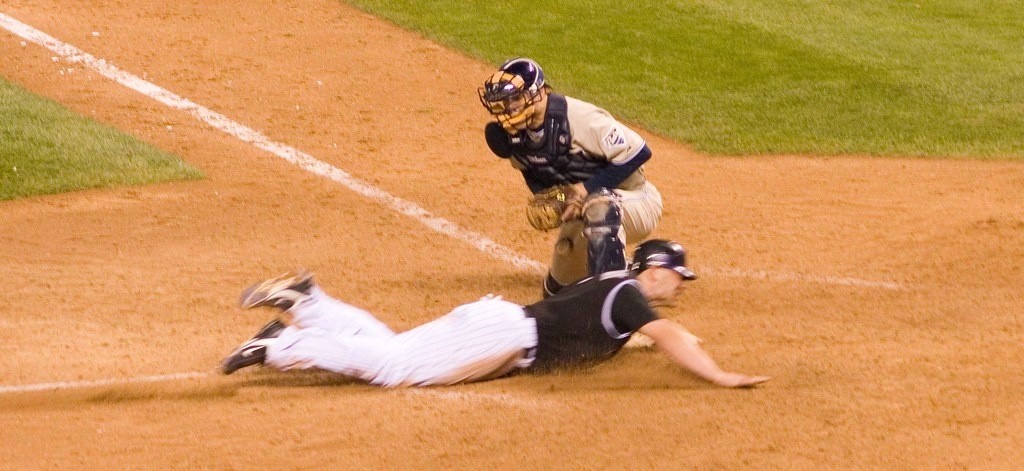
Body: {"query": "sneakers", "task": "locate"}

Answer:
[239,272,313,310]
[223,320,287,375]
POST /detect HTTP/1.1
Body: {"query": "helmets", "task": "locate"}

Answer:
[478,58,546,137]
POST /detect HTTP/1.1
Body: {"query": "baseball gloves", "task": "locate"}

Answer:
[525,181,579,234]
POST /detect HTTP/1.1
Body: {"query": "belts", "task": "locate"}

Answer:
[523,302,538,359]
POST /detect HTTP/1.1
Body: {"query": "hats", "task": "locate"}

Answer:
[631,239,696,280]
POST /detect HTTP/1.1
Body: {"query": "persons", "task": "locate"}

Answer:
[220,239,771,390]
[476,58,663,300]
